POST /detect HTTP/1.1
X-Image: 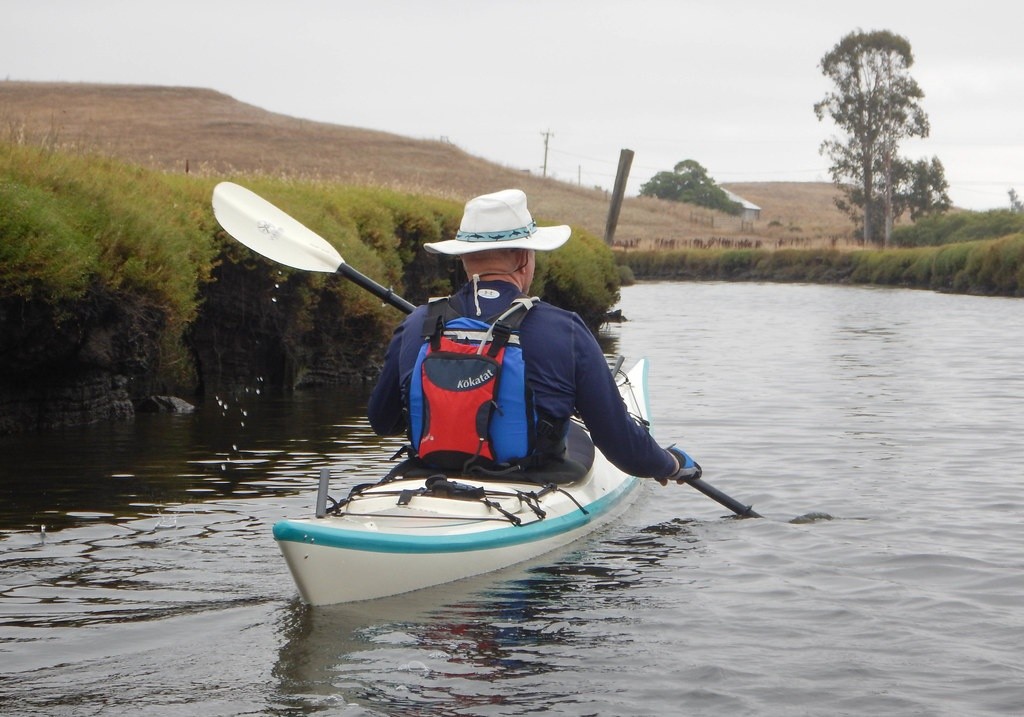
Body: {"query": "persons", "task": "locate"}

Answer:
[365,189,706,485]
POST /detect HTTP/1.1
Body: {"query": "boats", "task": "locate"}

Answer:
[268,356,653,610]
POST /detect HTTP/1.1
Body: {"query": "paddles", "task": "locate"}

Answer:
[207,180,764,518]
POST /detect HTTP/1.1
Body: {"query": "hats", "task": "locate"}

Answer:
[423,190,571,255]
